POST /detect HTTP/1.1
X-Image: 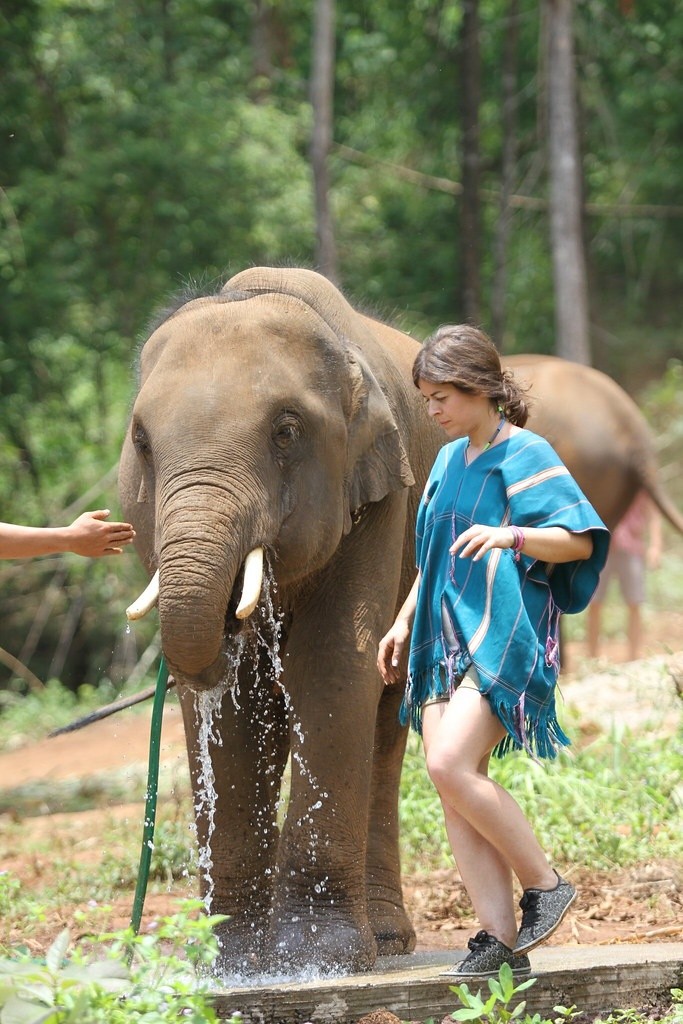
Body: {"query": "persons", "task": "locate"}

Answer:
[0,509,136,562]
[375,321,613,981]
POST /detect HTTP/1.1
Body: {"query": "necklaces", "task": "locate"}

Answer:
[482,405,506,453]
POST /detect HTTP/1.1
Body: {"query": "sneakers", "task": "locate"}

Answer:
[438,930,531,976]
[513,869,577,957]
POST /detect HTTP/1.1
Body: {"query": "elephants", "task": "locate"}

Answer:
[113,256,683,978]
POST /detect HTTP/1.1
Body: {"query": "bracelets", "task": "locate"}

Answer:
[509,524,525,562]
